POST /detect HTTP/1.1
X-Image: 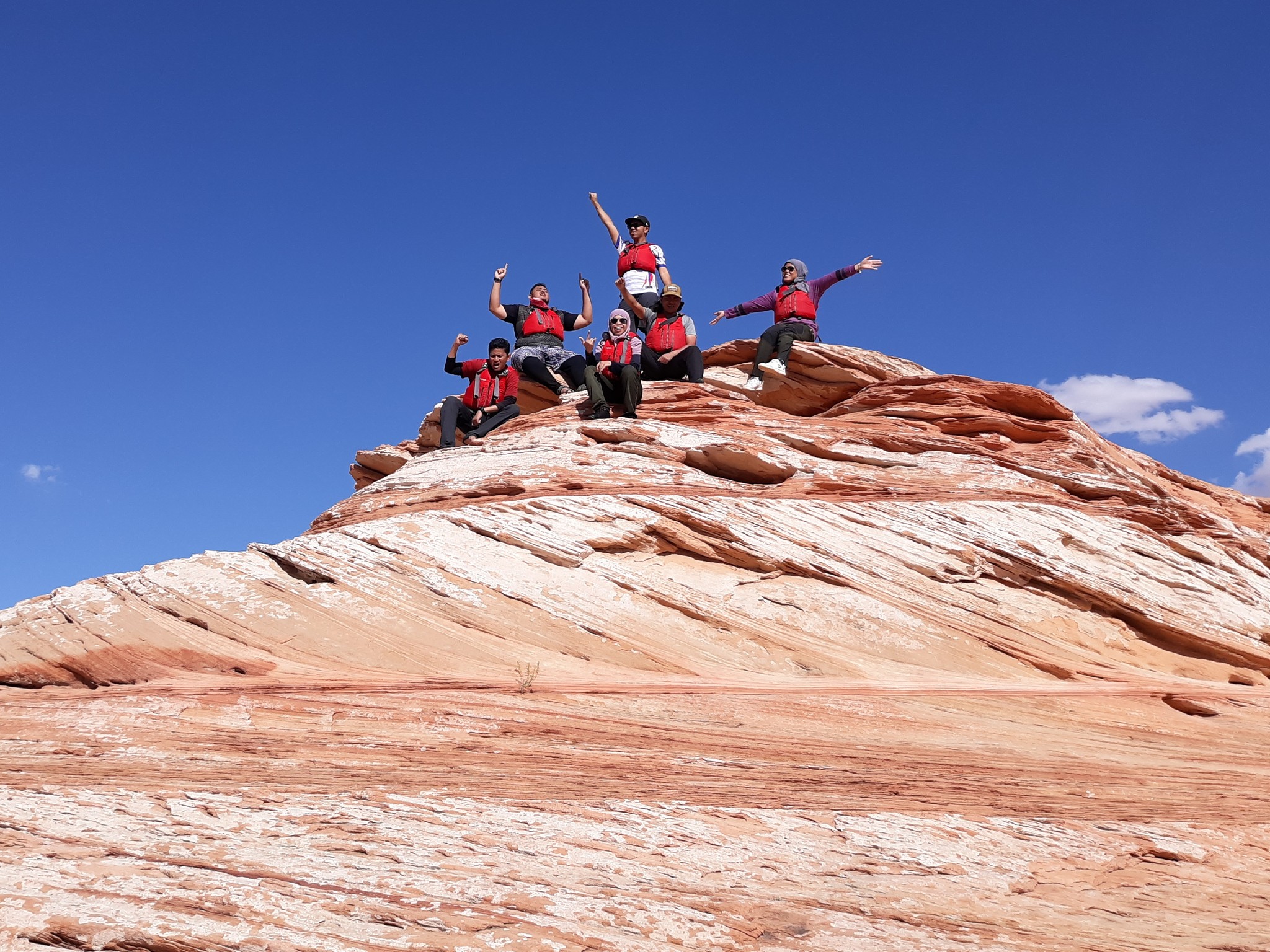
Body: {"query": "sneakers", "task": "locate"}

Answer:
[758,360,786,377]
[742,377,763,391]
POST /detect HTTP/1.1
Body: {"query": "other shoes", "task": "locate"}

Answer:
[622,412,638,419]
[587,406,610,420]
[576,384,587,391]
[564,387,574,393]
[464,435,484,446]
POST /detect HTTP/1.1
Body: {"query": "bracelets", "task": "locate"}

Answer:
[858,263,862,273]
[494,277,502,282]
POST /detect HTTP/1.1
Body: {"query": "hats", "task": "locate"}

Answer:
[625,215,650,229]
[661,283,683,298]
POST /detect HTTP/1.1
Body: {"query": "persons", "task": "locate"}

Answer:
[579,308,643,420]
[614,277,705,384]
[439,333,520,450]
[489,263,593,396]
[589,192,672,337]
[709,255,882,391]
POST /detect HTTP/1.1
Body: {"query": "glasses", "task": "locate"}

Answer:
[610,317,628,324]
[627,222,646,229]
[781,266,794,272]
